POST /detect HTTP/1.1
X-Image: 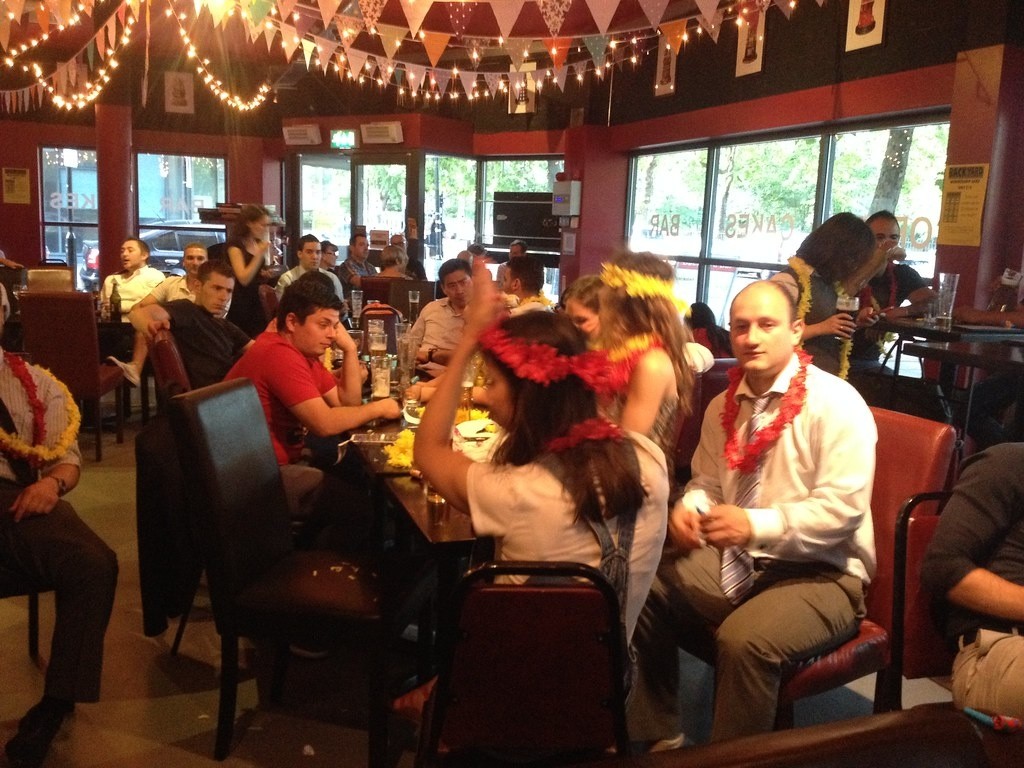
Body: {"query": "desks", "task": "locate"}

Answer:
[341,368,494,687]
[872,311,1024,464]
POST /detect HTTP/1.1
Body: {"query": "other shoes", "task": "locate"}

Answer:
[6,702,63,768]
[606,736,686,756]
[106,356,141,388]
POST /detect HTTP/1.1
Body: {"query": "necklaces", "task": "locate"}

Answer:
[520,290,551,306]
[550,417,622,453]
[718,346,813,474]
[0,351,81,467]
[787,255,853,380]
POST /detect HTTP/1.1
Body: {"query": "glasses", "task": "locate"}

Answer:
[323,251,335,256]
[392,242,404,250]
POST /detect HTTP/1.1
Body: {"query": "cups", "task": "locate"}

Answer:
[985,268,1024,313]
[409,290,419,327]
[348,319,422,428]
[923,271,959,333]
[424,483,445,506]
[834,296,859,342]
[352,290,363,329]
[456,364,476,423]
[879,239,907,262]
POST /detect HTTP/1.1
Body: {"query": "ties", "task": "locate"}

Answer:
[720,399,772,606]
[0,399,36,488]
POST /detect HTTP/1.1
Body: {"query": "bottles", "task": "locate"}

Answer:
[110,283,122,322]
[92,281,101,317]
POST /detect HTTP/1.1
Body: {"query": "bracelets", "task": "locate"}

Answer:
[428,351,433,361]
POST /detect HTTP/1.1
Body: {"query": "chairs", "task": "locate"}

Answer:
[361,560,630,768]
[663,402,968,731]
[17,267,129,460]
[359,276,401,357]
[147,323,428,768]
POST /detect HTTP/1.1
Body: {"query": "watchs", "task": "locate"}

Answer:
[51,474,67,495]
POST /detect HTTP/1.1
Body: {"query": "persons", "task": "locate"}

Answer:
[847,210,935,375]
[411,240,553,362]
[562,251,695,493]
[105,242,208,386]
[128,259,256,390]
[99,237,166,317]
[953,299,1024,329]
[684,302,734,359]
[625,280,879,753]
[321,235,427,299]
[921,442,1024,720]
[768,212,879,406]
[0,282,120,768]
[223,279,400,518]
[276,233,349,322]
[415,257,671,698]
[224,203,271,340]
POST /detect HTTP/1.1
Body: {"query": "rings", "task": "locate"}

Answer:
[840,326,843,330]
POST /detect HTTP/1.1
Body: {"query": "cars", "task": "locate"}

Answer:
[79,220,227,294]
